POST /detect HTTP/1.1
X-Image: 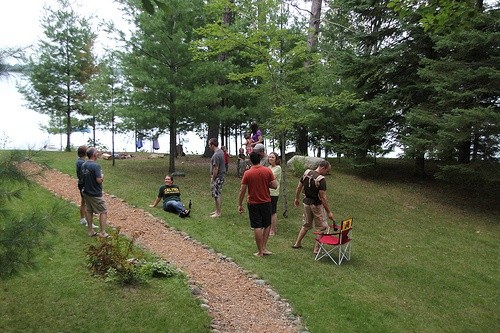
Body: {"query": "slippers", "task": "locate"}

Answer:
[105,234,112,238]
[88,232,99,236]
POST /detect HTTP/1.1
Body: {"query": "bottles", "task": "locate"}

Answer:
[189,200,192,209]
[333,220,338,231]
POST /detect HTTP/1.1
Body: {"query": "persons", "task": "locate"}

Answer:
[80,147,112,238]
[293,160,334,253]
[238,151,278,257]
[253,143,270,167]
[96,144,132,160]
[75,145,99,229]
[221,146,230,173]
[208,138,225,217]
[149,174,190,218]
[267,152,281,236]
[237,123,263,173]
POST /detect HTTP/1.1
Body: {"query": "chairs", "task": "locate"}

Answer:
[312,217,354,266]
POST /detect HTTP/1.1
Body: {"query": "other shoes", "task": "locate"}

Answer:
[80,218,88,224]
[185,210,190,217]
[179,212,186,217]
[87,222,99,228]
